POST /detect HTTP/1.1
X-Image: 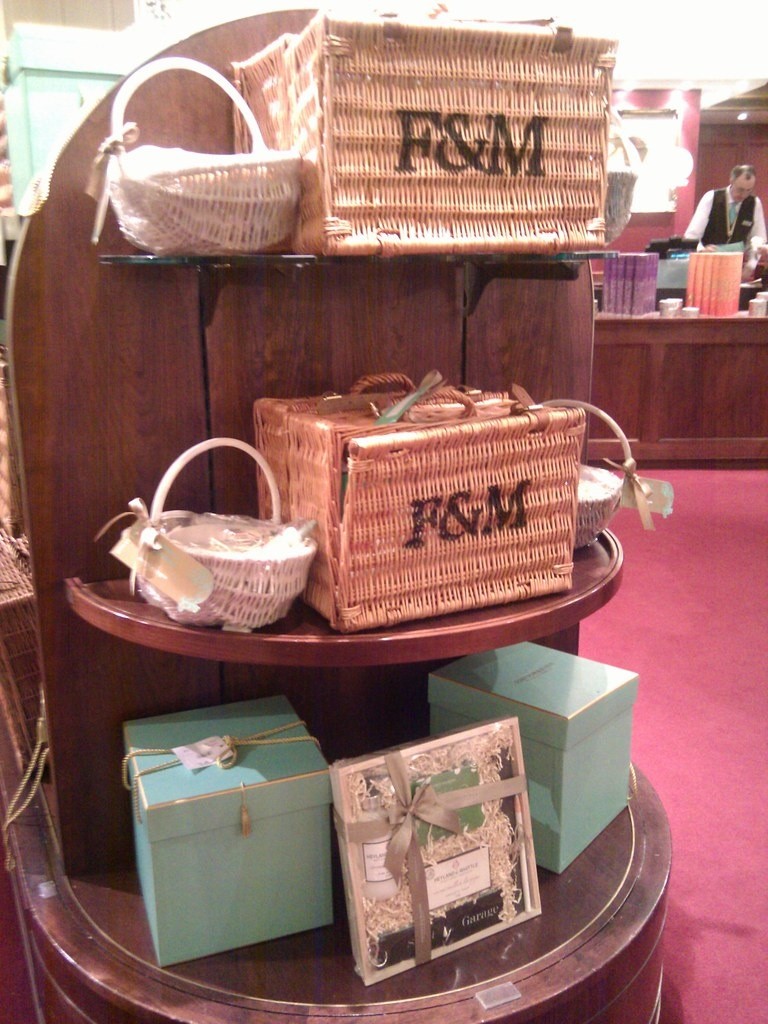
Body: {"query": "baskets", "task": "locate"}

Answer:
[536,398,637,551]
[228,9,614,257]
[128,434,320,631]
[602,106,638,249]
[83,57,299,257]
[251,368,582,632]
[0,514,48,775]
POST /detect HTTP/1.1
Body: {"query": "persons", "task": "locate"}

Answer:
[684,165,768,264]
